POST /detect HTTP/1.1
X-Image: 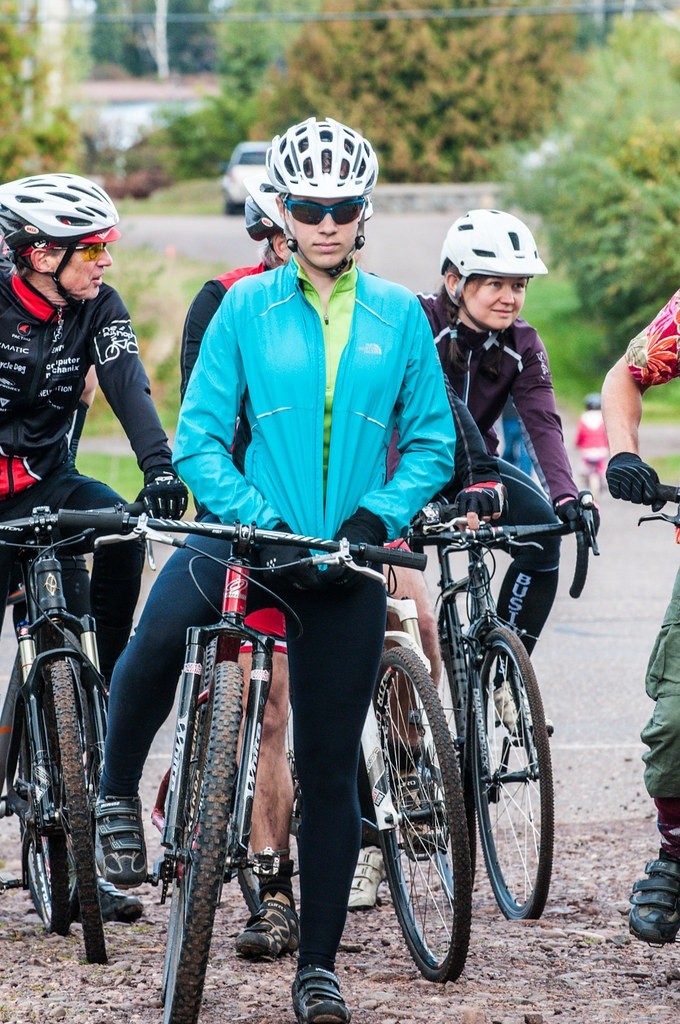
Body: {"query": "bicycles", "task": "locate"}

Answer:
[56,508,479,1024]
[408,488,604,923]
[0,500,149,965]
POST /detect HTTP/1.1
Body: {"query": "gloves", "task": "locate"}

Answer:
[133,467,189,519]
[319,507,388,593]
[258,522,319,594]
[554,494,600,547]
[606,452,666,513]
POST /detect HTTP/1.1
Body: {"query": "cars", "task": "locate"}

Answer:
[220,141,270,217]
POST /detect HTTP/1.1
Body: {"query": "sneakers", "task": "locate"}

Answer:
[68,875,144,924]
[346,845,387,912]
[93,793,148,888]
[291,965,352,1024]
[494,681,554,737]
[235,901,299,962]
[628,849,680,944]
[390,768,435,860]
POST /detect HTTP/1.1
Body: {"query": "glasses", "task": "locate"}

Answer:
[280,195,365,225]
[43,242,106,262]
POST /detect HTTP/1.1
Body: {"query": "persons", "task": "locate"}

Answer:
[95,114,458,1024]
[1,173,188,924]
[576,394,610,491]
[344,209,603,913]
[178,176,298,959]
[601,289,680,942]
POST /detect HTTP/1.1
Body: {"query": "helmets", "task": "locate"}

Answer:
[264,117,378,197]
[583,391,602,410]
[440,209,549,279]
[243,177,374,242]
[0,172,119,249]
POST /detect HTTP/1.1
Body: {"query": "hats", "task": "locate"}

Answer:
[17,218,121,257]
